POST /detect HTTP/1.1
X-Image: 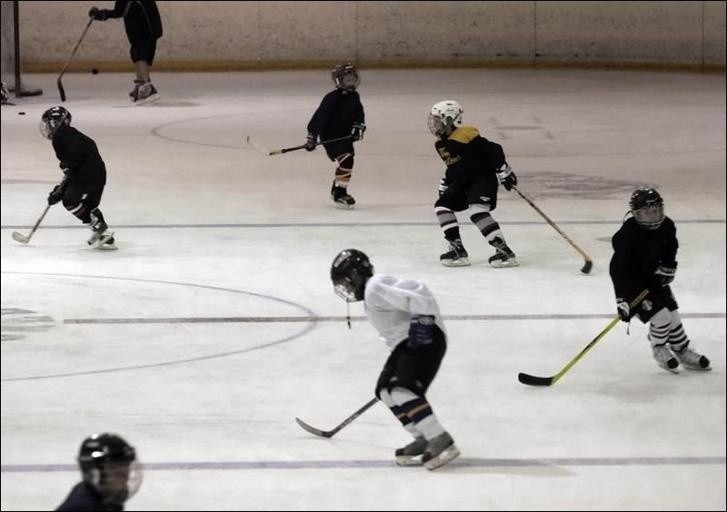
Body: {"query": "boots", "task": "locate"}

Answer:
[670,340,709,368]
[106,236,114,245]
[648,334,679,369]
[87,213,107,245]
[488,237,516,264]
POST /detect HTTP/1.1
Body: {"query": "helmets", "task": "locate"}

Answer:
[628,187,666,232]
[329,249,373,303]
[426,100,464,141]
[78,433,142,505]
[39,106,72,140]
[332,62,361,93]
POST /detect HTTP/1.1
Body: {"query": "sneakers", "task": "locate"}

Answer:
[422,431,453,462]
[129,77,157,102]
[330,181,355,205]
[396,434,427,456]
[440,237,468,259]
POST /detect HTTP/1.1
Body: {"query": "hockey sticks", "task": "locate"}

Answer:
[57,17,95,102]
[12,174,69,244]
[517,277,658,387]
[246,134,358,156]
[509,183,594,277]
[294,394,381,438]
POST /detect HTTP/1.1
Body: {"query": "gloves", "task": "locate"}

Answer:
[305,131,316,151]
[406,315,434,352]
[351,121,366,142]
[495,161,517,191]
[89,6,110,21]
[48,180,67,206]
[439,178,449,197]
[617,295,632,322]
[649,259,678,288]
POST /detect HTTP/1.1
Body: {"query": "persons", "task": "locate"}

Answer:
[427,99,519,267]
[610,187,710,373]
[40,105,117,251]
[87,1,163,102]
[56,432,145,512]
[328,250,461,473]
[304,61,367,206]
